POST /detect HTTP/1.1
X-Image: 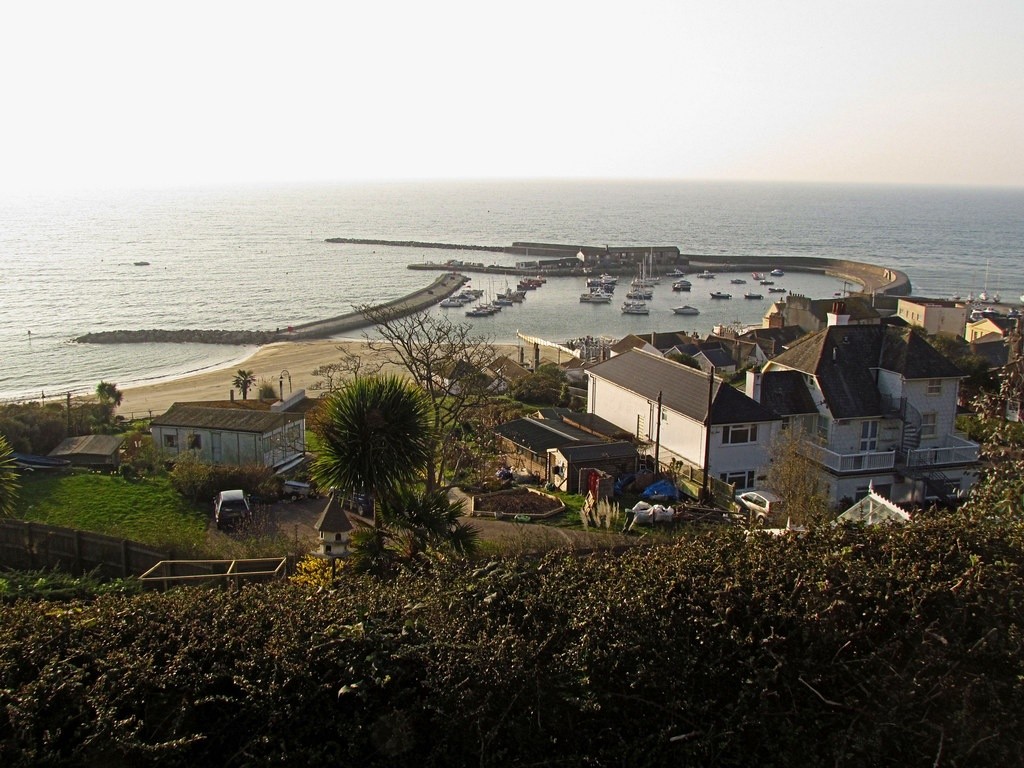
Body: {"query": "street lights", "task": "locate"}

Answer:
[279,375,286,402]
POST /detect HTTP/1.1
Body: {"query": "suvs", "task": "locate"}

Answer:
[212,489,253,530]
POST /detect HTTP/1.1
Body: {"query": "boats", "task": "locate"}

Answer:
[772,269,785,276]
[670,304,700,316]
[743,292,765,300]
[439,274,546,318]
[759,280,775,285]
[708,291,733,298]
[751,272,767,280]
[768,287,787,293]
[578,273,618,304]
[953,255,1022,322]
[697,270,716,278]
[730,278,746,283]
[672,279,692,291]
[665,268,685,277]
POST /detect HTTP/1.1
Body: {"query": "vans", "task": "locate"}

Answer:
[736,490,787,529]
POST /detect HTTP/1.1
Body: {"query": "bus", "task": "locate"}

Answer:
[538,256,580,269]
[515,260,538,270]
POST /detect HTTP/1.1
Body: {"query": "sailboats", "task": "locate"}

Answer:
[621,247,663,315]
